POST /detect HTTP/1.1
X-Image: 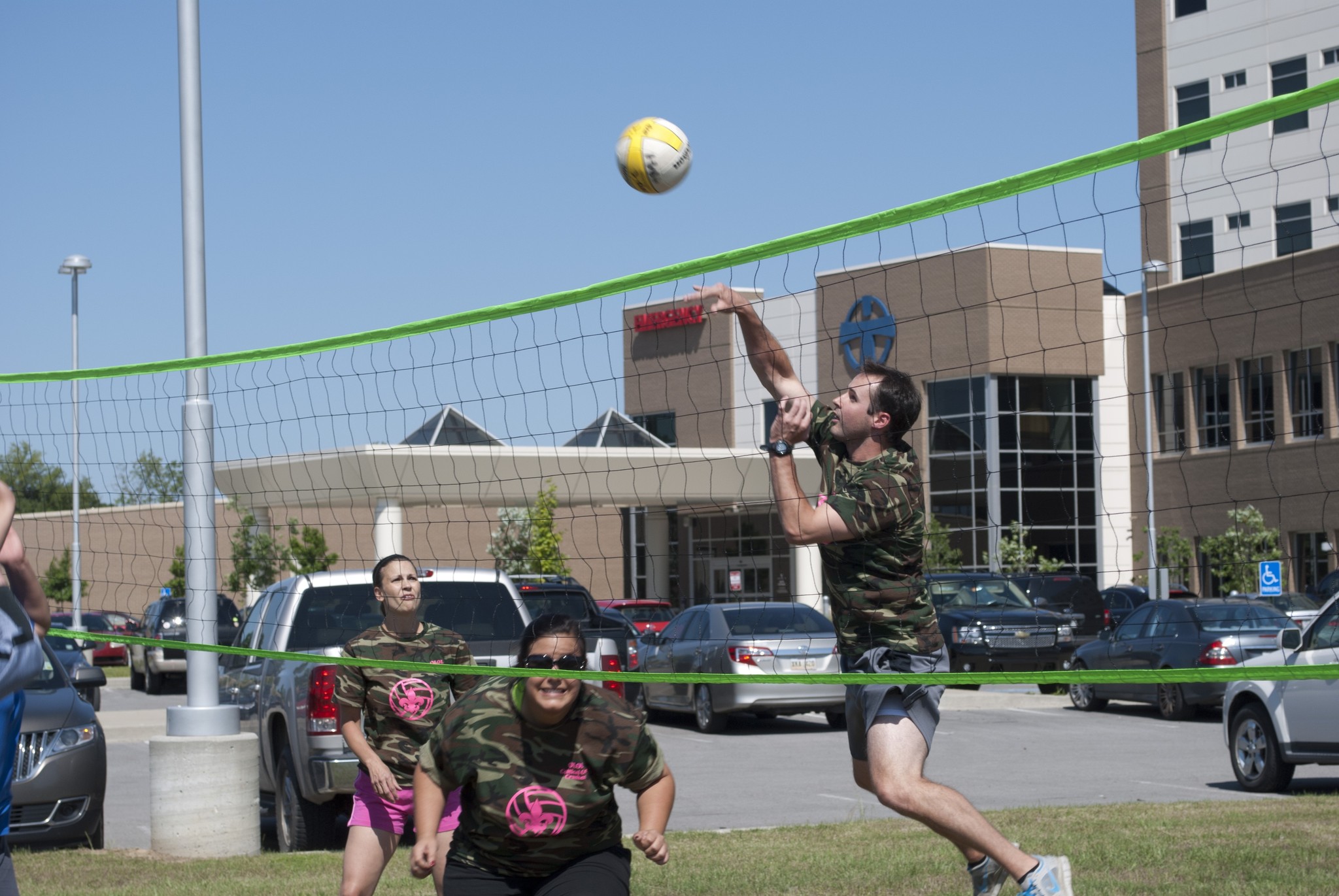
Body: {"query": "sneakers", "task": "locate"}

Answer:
[967,841,1021,896]
[1019,854,1073,896]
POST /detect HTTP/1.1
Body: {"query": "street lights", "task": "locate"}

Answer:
[1139,260,1169,570]
[56,254,93,646]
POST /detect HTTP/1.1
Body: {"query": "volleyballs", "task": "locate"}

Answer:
[616,117,693,195]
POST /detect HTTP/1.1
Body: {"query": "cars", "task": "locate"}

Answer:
[1065,598,1306,720]
[1233,591,1339,645]
[29,610,143,711]
[634,601,850,733]
[1222,592,1339,791]
[8,636,109,849]
[1304,569,1339,612]
[1097,582,1198,629]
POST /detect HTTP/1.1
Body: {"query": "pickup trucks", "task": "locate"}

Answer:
[216,568,627,858]
[423,571,645,704]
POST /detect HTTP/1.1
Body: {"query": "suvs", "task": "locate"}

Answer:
[924,572,1079,695]
[125,592,246,693]
[594,600,674,638]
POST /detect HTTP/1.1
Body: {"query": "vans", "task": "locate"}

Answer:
[1004,572,1112,640]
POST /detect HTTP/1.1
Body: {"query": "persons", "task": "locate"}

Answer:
[684,284,1072,896]
[333,553,479,896]
[410,615,675,896]
[0,481,49,896]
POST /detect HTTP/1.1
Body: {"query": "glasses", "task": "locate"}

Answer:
[521,653,587,672]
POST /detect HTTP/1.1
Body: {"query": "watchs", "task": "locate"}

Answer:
[758,441,794,457]
